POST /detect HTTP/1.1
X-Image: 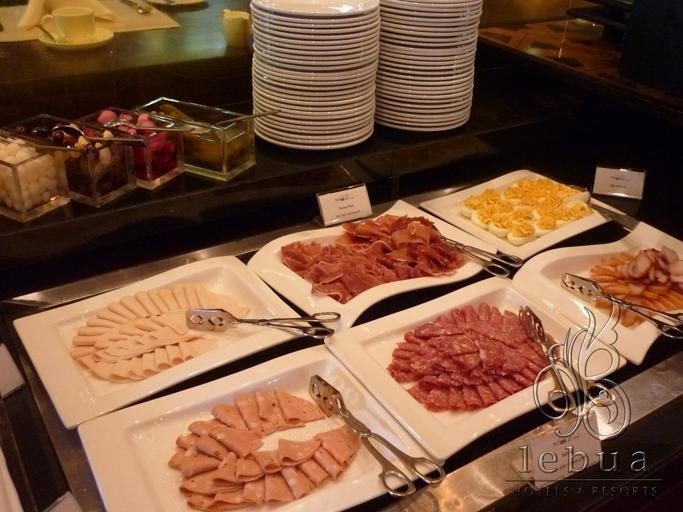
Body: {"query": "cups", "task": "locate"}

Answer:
[221,9,251,49]
[41,6,96,42]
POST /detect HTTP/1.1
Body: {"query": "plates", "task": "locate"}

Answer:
[376,0,482,133]
[512,221,683,365]
[420,169,628,261]
[38,26,115,52]
[250,0,380,150]
[77,343,444,512]
[248,198,498,337]
[324,275,628,460]
[12,254,316,429]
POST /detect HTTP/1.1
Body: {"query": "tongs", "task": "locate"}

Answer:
[308,375,446,498]
[560,273,683,340]
[185,308,340,338]
[519,306,616,414]
[424,222,523,279]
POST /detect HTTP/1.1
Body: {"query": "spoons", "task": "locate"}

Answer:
[567,184,640,231]
[105,118,193,134]
[187,110,281,130]
[51,123,148,148]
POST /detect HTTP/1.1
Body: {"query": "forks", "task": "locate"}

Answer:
[121,0,154,15]
